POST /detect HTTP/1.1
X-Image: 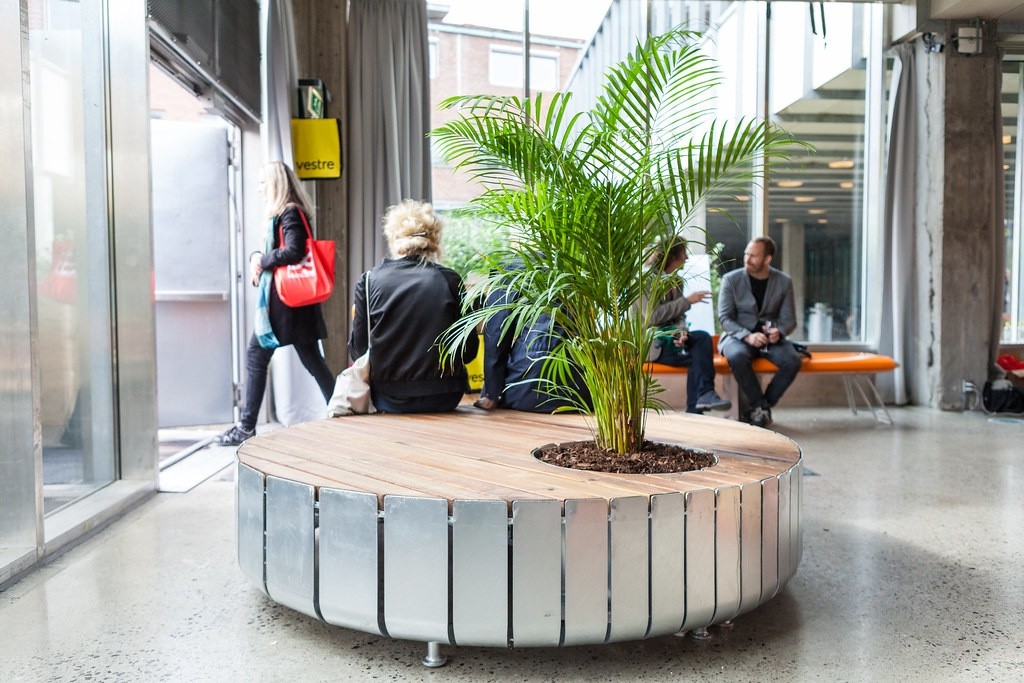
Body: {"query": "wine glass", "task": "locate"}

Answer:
[760,321,777,353]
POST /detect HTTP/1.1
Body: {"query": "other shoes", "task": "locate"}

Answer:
[696,391,732,411]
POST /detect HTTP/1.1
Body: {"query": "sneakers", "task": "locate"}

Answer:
[749,406,772,427]
[214,425,255,446]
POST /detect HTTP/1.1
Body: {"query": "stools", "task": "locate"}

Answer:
[643,352,741,423]
[742,351,900,424]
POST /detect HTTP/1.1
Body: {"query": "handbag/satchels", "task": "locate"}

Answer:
[275,205,336,307]
[324,351,371,419]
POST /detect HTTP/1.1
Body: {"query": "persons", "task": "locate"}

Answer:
[632,235,732,416]
[348,200,479,415]
[476,247,598,415]
[211,162,335,447]
[717,236,802,424]
